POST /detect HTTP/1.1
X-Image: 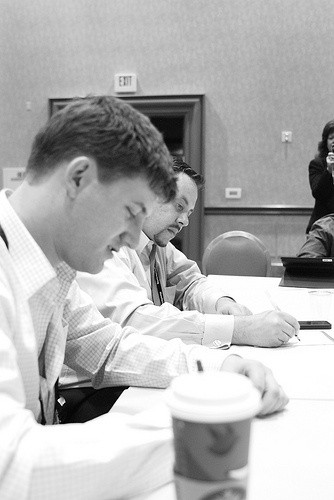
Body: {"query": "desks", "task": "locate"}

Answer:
[111,272,334,500]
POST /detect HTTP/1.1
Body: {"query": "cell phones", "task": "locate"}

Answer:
[298,321,331,329]
[331,144,334,153]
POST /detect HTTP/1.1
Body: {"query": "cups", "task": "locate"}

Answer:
[160,370,263,500]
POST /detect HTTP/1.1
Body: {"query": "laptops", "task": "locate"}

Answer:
[278,257,334,289]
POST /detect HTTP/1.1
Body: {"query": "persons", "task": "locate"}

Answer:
[55,118,334,424]
[0,94,290,500]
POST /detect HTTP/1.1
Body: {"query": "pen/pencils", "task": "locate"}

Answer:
[274,301,302,341]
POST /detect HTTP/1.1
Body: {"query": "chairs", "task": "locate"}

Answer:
[202,230,270,277]
